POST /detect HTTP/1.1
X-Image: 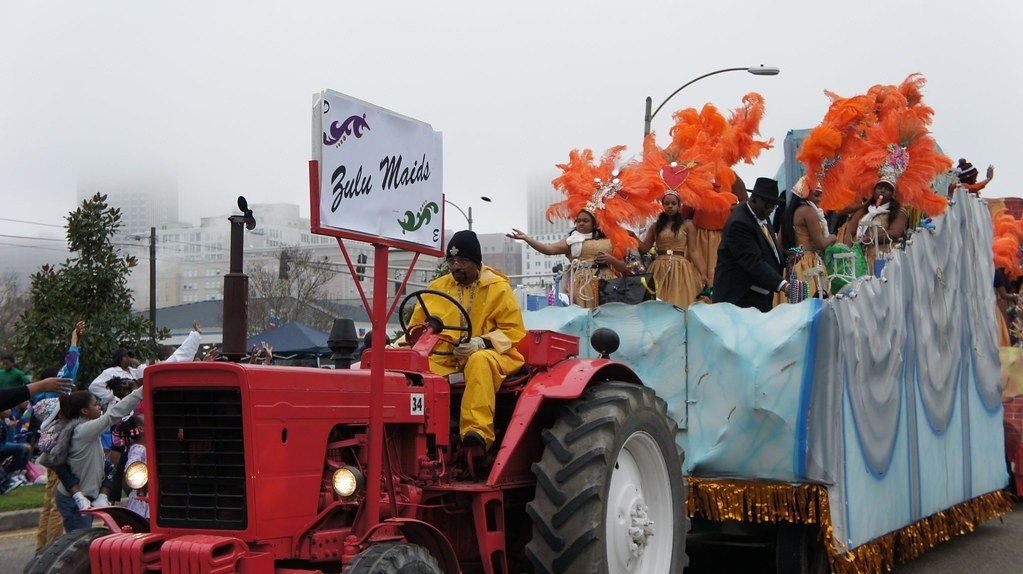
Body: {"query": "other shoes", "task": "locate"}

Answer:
[5,475,32,492]
[463,431,487,468]
[92,497,110,507]
[76,497,91,517]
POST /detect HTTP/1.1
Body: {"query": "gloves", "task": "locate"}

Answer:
[453,335,487,358]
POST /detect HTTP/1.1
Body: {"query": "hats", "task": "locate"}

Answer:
[745,177,786,203]
[446,230,482,264]
[110,349,135,365]
[957,159,978,179]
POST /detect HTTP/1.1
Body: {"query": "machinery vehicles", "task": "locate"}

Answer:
[22,195,692,574]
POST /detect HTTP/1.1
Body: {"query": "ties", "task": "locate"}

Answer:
[761,224,775,253]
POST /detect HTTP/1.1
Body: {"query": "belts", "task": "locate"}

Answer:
[657,250,684,256]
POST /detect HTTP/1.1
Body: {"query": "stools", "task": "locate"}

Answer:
[451,366,540,387]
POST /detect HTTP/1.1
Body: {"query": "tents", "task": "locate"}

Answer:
[220,321,364,367]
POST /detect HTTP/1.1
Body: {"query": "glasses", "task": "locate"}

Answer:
[765,202,779,210]
[447,257,469,268]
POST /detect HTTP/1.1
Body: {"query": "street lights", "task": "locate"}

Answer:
[644,63,781,141]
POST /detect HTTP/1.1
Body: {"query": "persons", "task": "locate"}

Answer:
[0,319,273,532]
[392,230,528,450]
[780,176,834,306]
[628,192,709,307]
[834,170,907,271]
[948,157,993,191]
[998,264,1023,347]
[711,177,810,310]
[505,209,626,311]
